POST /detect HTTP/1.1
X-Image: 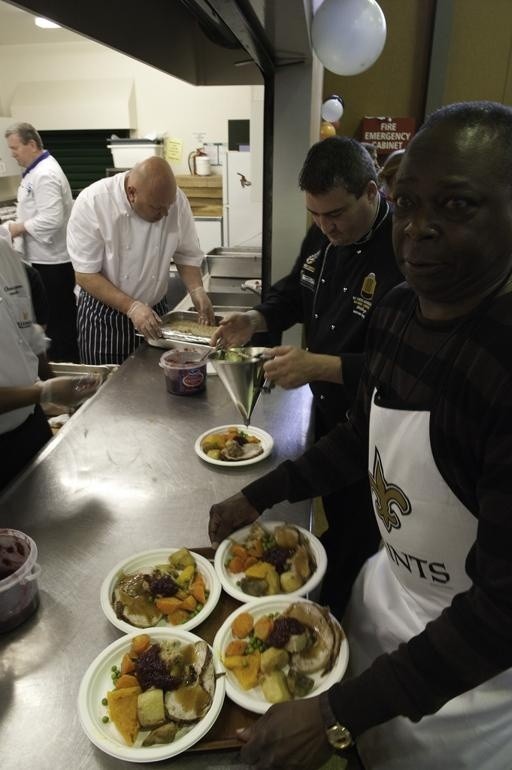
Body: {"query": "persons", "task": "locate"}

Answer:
[0,123,80,381]
[378,148,407,196]
[64,156,216,366]
[362,143,381,173]
[208,134,405,596]
[207,97,511,770]
[0,237,101,493]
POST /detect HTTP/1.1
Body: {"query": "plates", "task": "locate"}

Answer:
[194,424,275,467]
[76,626,227,762]
[212,593,352,715]
[212,518,330,604]
[99,546,221,637]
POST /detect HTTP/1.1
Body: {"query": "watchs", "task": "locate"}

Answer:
[319,689,357,759]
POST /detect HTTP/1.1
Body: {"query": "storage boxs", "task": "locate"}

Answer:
[106,138,163,169]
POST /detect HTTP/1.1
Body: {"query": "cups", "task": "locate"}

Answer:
[195,156,212,176]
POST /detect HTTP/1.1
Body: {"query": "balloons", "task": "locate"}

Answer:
[310,0,388,77]
[321,96,344,122]
[319,122,337,140]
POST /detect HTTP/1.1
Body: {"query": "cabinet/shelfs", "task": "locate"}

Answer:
[168,216,223,278]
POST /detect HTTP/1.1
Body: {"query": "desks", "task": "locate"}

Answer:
[1,339,315,770]
